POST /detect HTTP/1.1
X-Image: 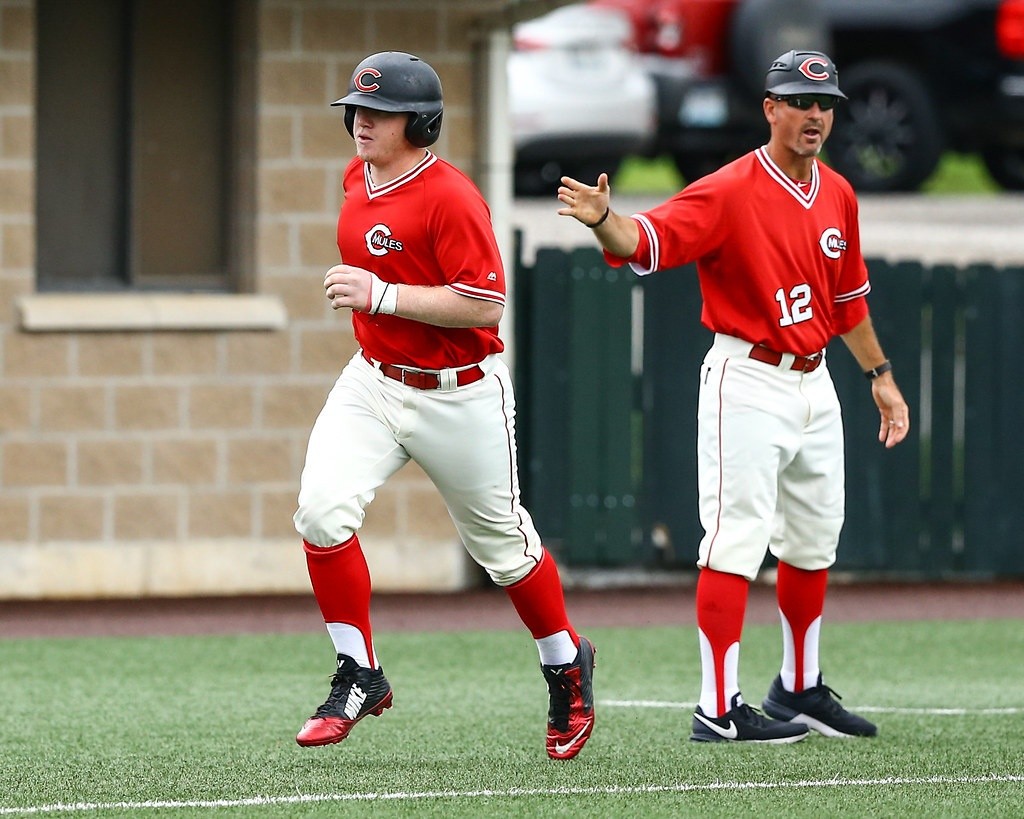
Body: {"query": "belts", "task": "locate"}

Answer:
[749,342,824,374]
[362,352,483,391]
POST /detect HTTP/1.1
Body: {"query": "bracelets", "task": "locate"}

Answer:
[586,206,609,228]
[864,359,892,380]
[358,273,398,316]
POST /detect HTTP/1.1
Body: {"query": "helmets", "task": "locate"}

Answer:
[329,51,444,148]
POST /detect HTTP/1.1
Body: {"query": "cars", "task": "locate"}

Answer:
[506,4,657,200]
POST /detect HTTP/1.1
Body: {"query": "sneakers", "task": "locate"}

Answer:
[540,635,597,760]
[763,675,877,738]
[295,654,392,747]
[692,704,809,747]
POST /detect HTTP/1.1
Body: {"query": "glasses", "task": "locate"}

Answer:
[767,93,836,110]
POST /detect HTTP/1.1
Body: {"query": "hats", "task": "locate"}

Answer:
[764,50,848,99]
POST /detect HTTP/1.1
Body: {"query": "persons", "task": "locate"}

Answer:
[291,51,598,761]
[554,49,910,745]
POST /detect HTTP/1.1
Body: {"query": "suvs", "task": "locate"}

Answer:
[589,0,1024,198]
